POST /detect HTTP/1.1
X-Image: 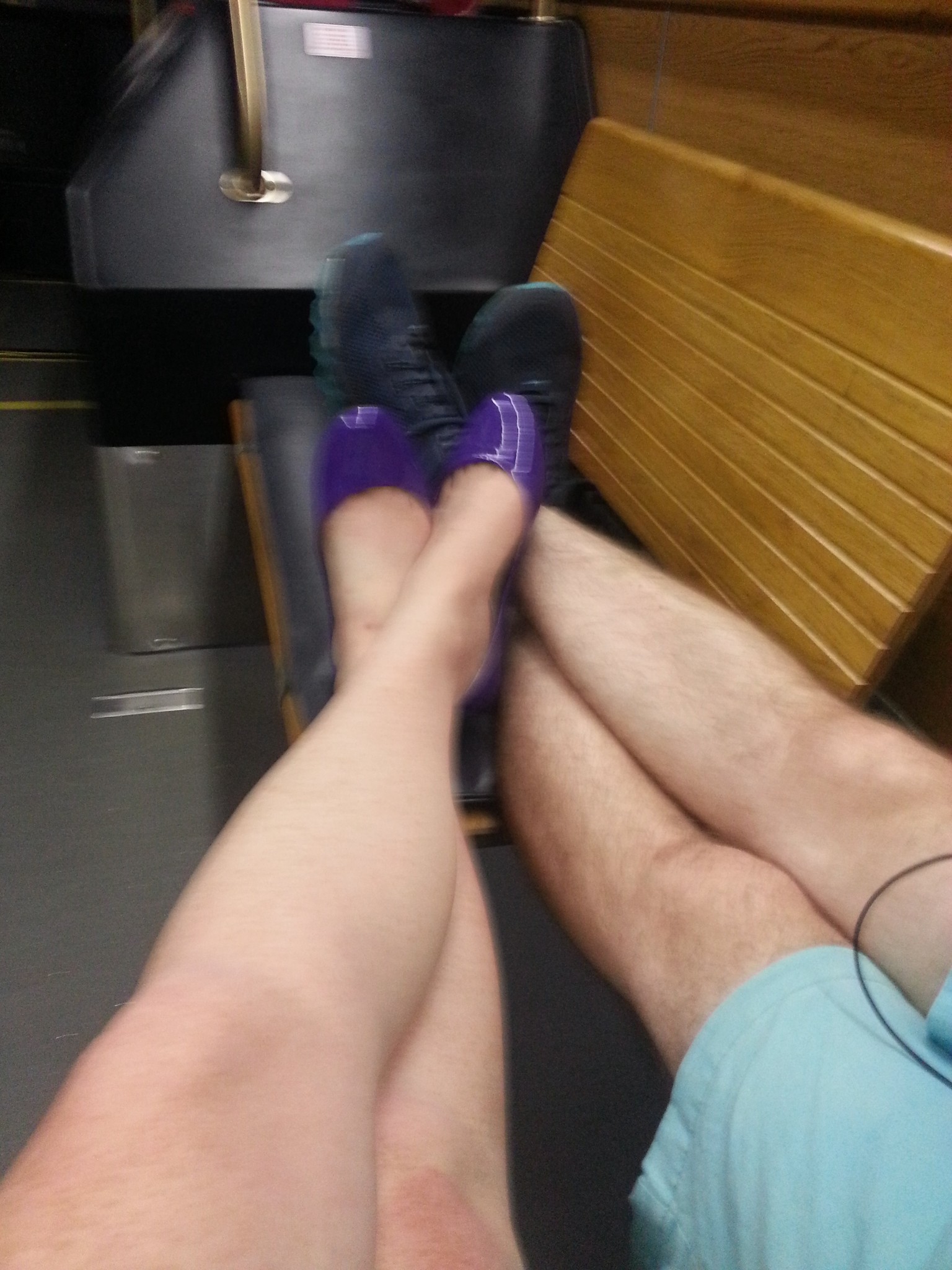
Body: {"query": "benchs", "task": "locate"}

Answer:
[229,118,952,849]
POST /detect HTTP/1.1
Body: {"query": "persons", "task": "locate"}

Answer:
[0,386,530,1270]
[317,234,951,1270]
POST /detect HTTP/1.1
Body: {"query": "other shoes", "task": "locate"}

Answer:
[434,282,583,512]
[312,233,480,475]
[452,390,544,712]
[304,405,436,693]
